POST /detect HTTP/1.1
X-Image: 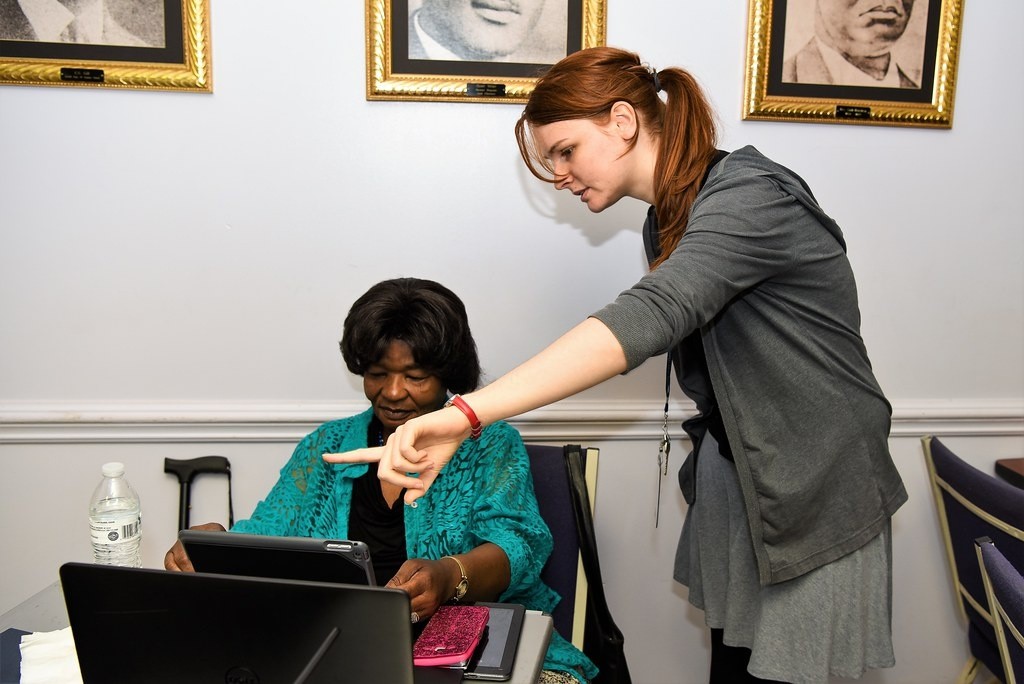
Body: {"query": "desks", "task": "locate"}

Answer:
[0,577,553,684]
[995,459,1024,490]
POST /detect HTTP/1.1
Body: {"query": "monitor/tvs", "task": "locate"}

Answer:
[59,563,415,684]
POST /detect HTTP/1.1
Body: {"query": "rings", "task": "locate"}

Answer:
[410,611,420,624]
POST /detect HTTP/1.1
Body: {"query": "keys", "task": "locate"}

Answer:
[663,434,671,476]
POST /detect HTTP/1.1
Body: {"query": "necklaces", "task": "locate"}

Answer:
[378,430,385,446]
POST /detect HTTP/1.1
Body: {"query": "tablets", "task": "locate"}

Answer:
[177,529,377,588]
[462,602,526,681]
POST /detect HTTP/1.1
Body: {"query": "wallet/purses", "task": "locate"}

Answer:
[412,603,489,666]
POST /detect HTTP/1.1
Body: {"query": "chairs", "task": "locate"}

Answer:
[519,444,599,661]
[973,536,1024,684]
[920,436,1024,684]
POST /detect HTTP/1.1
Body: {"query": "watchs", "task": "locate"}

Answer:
[443,393,483,440]
[440,556,469,602]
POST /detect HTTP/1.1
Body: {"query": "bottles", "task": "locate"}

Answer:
[88,463,142,568]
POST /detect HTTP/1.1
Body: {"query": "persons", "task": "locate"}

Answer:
[164,278,601,683]
[0,0,165,48]
[320,46,910,684]
[782,0,921,90]
[407,0,568,63]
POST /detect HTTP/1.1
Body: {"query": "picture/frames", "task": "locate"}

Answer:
[365,0,610,105]
[742,1,965,130]
[0,0,215,94]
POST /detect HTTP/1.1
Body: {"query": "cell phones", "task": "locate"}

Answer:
[411,607,490,670]
